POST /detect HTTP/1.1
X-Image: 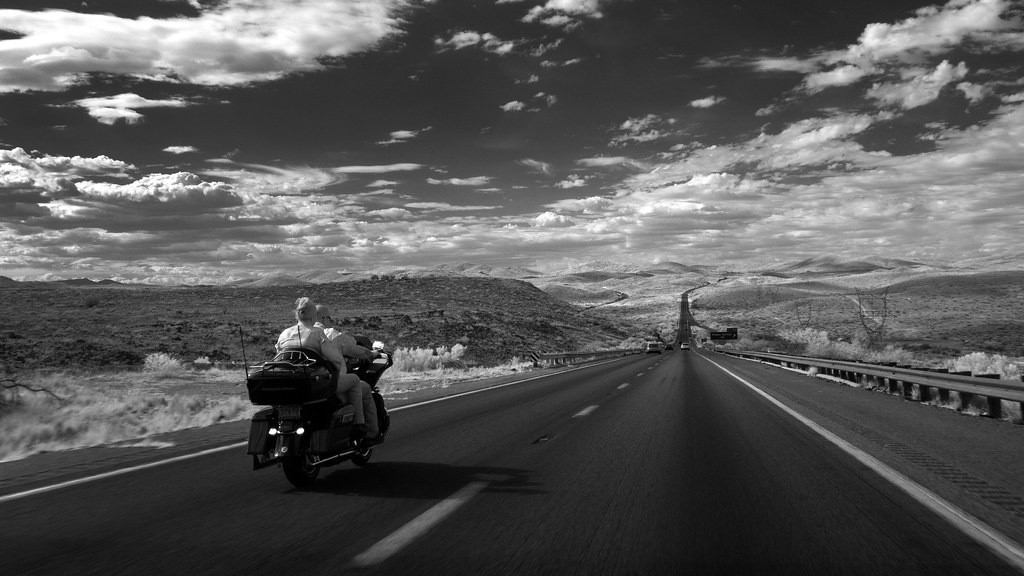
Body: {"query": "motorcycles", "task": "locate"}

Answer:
[237,324,393,488]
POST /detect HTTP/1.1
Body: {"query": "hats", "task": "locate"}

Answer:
[296,297,316,320]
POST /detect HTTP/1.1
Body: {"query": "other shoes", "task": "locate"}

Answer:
[351,424,367,439]
[362,432,384,447]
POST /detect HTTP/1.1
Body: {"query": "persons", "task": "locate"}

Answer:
[277,296,383,446]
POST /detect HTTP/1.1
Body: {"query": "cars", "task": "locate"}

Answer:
[646,342,662,354]
[680,343,691,351]
[664,344,673,351]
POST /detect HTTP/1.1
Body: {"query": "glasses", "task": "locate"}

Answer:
[321,316,332,320]
